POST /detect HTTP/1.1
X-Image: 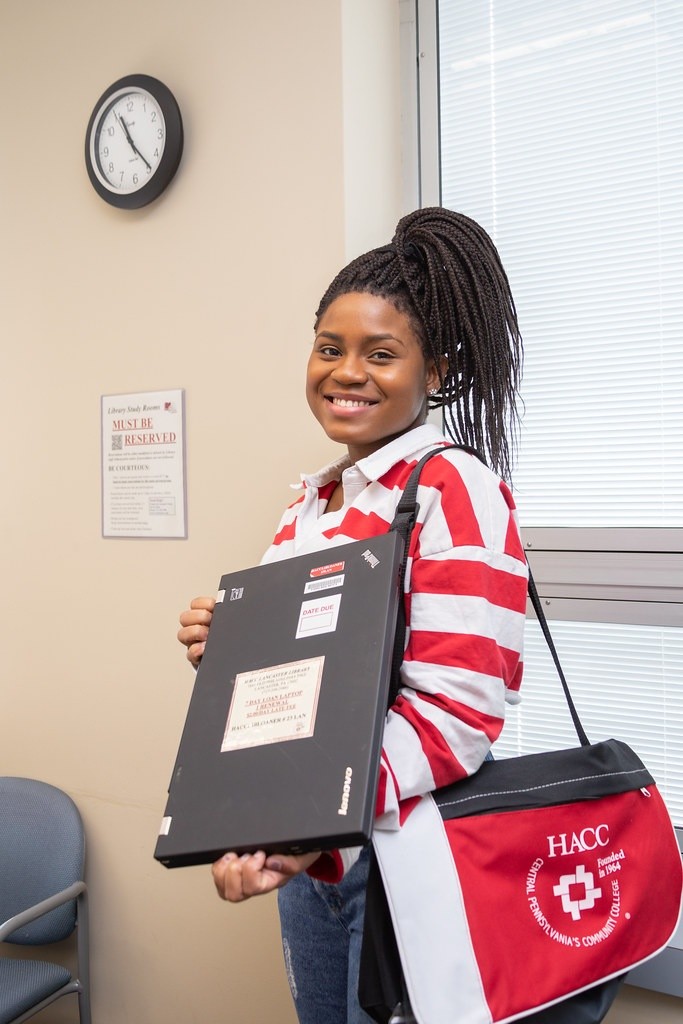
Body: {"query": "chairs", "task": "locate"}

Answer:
[0,775,91,1024]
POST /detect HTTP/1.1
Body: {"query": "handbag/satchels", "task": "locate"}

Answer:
[359,737,683,1024]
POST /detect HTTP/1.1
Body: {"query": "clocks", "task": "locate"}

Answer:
[85,74,184,211]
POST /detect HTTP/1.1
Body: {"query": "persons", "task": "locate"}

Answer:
[177,208,530,1024]
